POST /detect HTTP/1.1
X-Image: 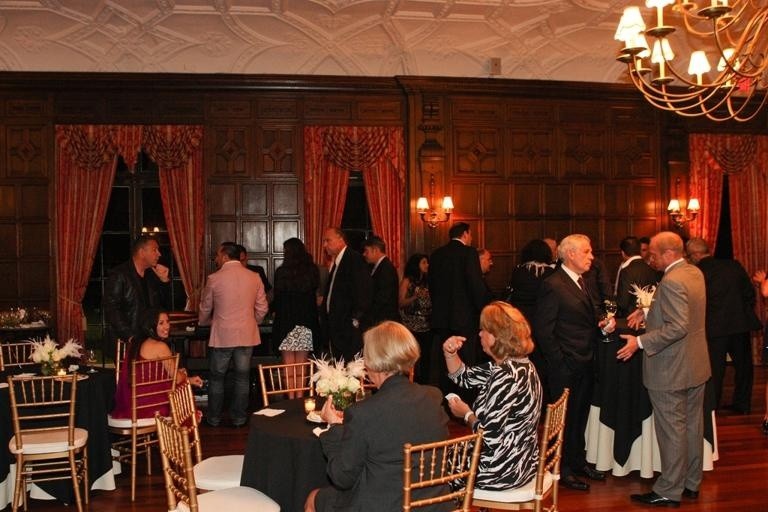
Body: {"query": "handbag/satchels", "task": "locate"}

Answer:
[500,284,516,302]
[403,296,421,314]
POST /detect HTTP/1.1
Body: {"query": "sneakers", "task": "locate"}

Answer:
[232,417,246,426]
[207,413,219,425]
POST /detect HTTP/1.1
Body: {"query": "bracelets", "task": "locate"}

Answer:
[445,353,458,359]
[463,411,474,423]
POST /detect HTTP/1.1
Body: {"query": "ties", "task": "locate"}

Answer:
[578,277,589,297]
[321,264,337,313]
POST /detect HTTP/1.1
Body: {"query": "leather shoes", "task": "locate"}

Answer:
[575,465,606,480]
[629,492,680,507]
[682,489,699,498]
[724,403,750,415]
[560,476,590,489]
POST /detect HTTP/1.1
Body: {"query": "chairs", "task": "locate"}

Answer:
[165,377,167,381]
[8,373,93,509]
[107,334,282,511]
[404,388,571,512]
[0,340,44,373]
[256,360,314,405]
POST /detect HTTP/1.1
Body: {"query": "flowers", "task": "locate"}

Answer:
[631,282,659,309]
[30,335,82,376]
[313,356,362,407]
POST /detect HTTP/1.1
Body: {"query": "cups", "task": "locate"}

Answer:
[604,300,617,317]
[304,398,316,414]
[57,369,66,378]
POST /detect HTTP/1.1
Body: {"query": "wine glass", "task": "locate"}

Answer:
[600,319,617,343]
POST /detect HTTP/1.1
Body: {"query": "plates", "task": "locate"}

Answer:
[56,372,90,381]
[306,409,348,425]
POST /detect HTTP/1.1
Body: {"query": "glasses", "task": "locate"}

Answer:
[686,251,701,258]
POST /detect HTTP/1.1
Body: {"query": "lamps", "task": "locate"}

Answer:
[608,1,768,126]
[414,195,455,227]
[669,197,704,229]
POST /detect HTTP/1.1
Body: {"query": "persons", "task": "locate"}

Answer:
[441,300,556,503]
[113,221,755,432]
[304,319,457,510]
[533,232,617,491]
[620,231,718,504]
